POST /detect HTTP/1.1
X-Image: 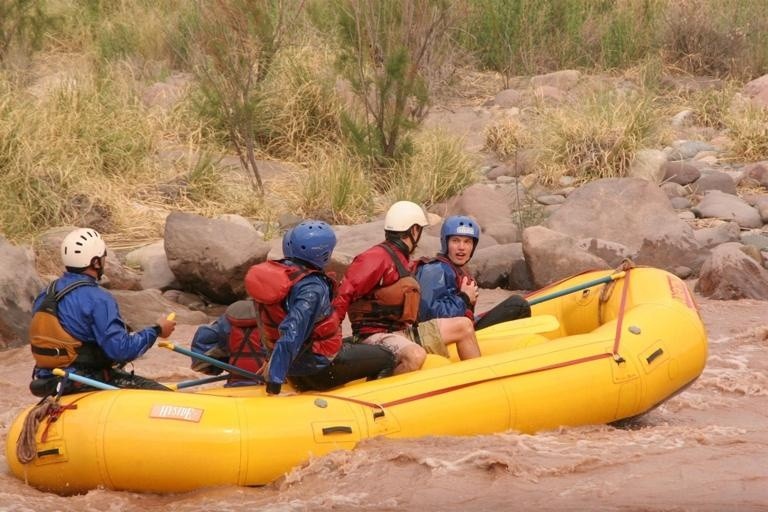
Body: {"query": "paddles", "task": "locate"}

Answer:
[474,271,626,331]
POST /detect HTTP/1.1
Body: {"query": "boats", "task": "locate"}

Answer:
[7,260,707,493]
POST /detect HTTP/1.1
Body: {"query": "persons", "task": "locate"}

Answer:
[242,220,395,396]
[414,215,480,326]
[329,201,482,380]
[26,229,176,392]
[192,296,281,389]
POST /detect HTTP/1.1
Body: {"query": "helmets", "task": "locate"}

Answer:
[61,229,108,270]
[293,220,336,269]
[386,201,428,232]
[282,230,294,258]
[442,217,479,256]
